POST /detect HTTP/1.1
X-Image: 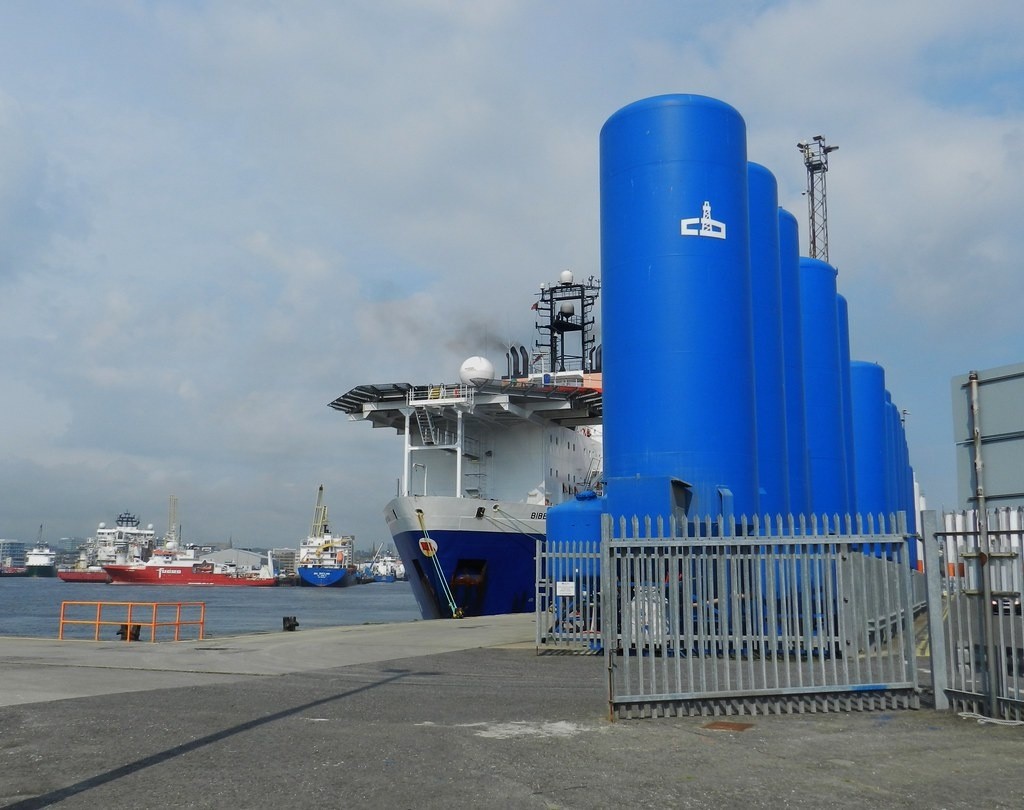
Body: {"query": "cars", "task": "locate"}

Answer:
[989,597,1021,615]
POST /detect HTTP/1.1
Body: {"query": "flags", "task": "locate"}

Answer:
[531,303,538,310]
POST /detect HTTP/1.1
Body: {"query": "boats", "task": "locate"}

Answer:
[326,270,604,620]
[362,564,399,584]
[100,508,279,586]
[294,484,358,587]
[0,525,57,578]
[58,518,155,583]
